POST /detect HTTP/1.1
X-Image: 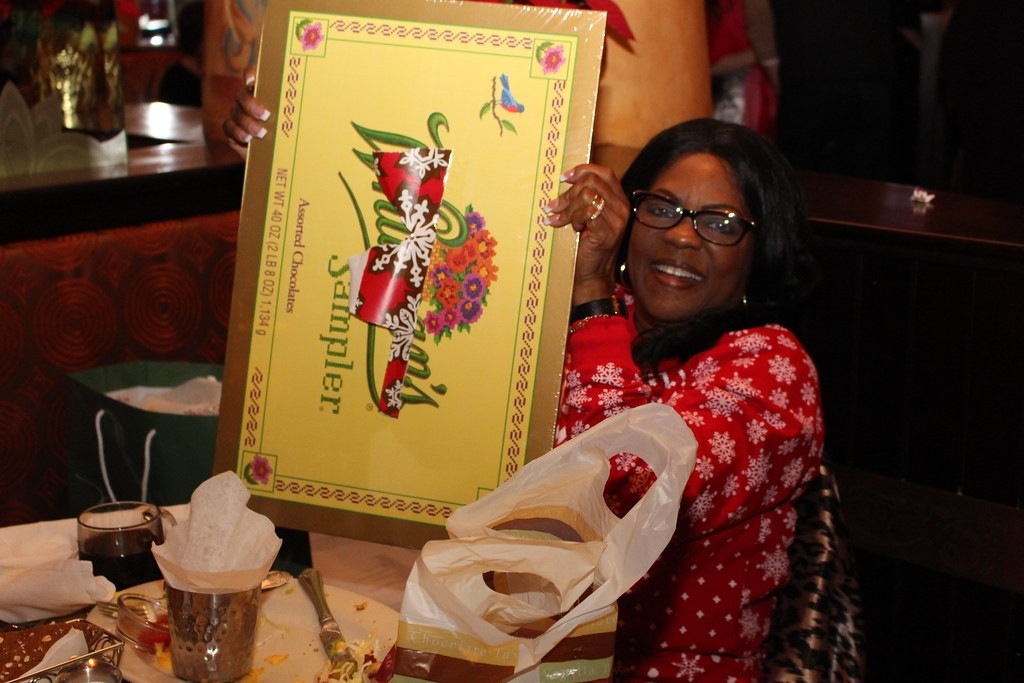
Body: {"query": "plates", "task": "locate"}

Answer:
[89,579,400,683]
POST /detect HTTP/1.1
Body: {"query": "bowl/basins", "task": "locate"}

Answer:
[118,592,171,654]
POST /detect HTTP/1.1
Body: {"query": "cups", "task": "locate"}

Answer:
[77,501,177,588]
[164,581,261,683]
[56,659,123,683]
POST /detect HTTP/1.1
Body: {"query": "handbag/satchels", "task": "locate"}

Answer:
[372,402,701,683]
[66,358,224,519]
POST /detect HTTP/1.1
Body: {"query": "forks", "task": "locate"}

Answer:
[97,570,286,619]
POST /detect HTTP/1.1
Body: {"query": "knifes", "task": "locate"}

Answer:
[300,570,364,683]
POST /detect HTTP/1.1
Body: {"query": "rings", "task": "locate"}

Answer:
[591,193,606,211]
[585,210,601,224]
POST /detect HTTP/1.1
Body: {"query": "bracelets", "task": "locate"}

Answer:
[569,295,629,326]
[567,314,610,334]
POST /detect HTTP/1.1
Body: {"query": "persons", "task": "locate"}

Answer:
[223,64,825,682]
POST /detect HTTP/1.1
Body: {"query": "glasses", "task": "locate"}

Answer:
[629,190,764,248]
